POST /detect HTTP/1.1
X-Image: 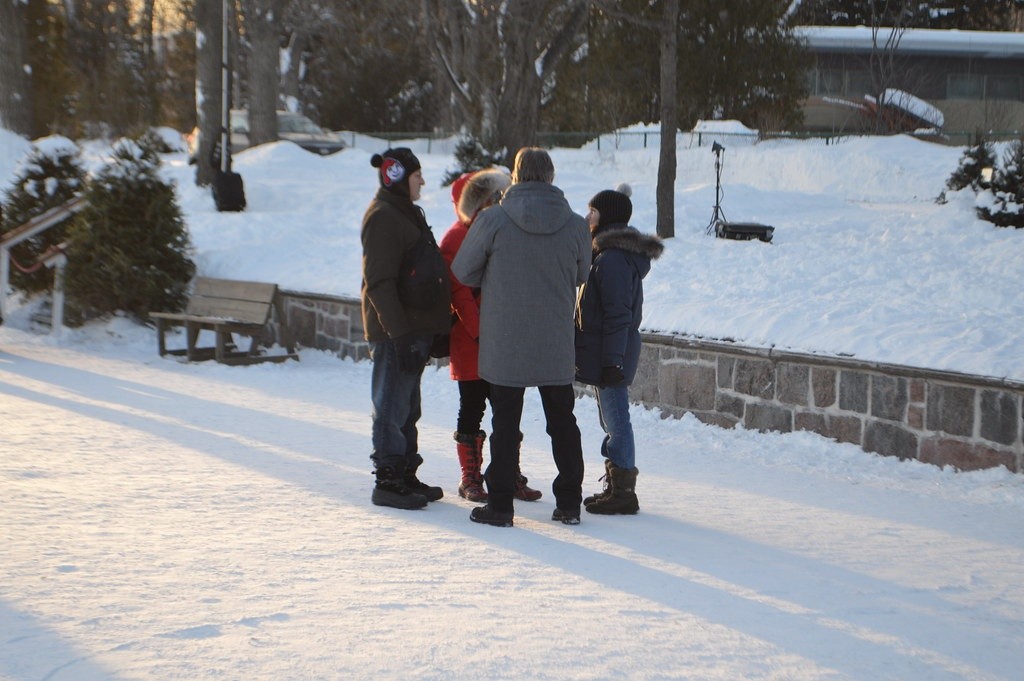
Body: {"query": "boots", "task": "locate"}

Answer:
[370,453,427,510]
[585,465,639,515]
[513,430,542,502]
[402,452,443,502]
[454,429,488,504]
[583,458,612,506]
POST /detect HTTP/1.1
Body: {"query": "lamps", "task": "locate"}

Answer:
[711,140,726,153]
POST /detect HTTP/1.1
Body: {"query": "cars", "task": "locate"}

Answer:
[188,107,345,165]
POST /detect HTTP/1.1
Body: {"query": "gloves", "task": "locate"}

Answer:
[393,332,424,376]
[601,366,624,389]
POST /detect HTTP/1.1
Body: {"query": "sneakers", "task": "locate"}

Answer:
[470,506,514,528]
[552,505,581,525]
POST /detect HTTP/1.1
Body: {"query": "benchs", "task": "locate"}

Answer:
[147,276,300,366]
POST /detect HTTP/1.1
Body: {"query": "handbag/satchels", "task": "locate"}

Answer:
[430,313,457,358]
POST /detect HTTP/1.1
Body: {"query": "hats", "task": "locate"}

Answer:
[371,148,421,198]
[589,182,632,240]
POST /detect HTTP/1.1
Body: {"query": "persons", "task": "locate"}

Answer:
[361,147,451,508]
[450,149,591,526]
[575,184,664,514]
[439,169,542,502]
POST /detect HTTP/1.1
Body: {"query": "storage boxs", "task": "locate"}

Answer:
[715,222,775,245]
